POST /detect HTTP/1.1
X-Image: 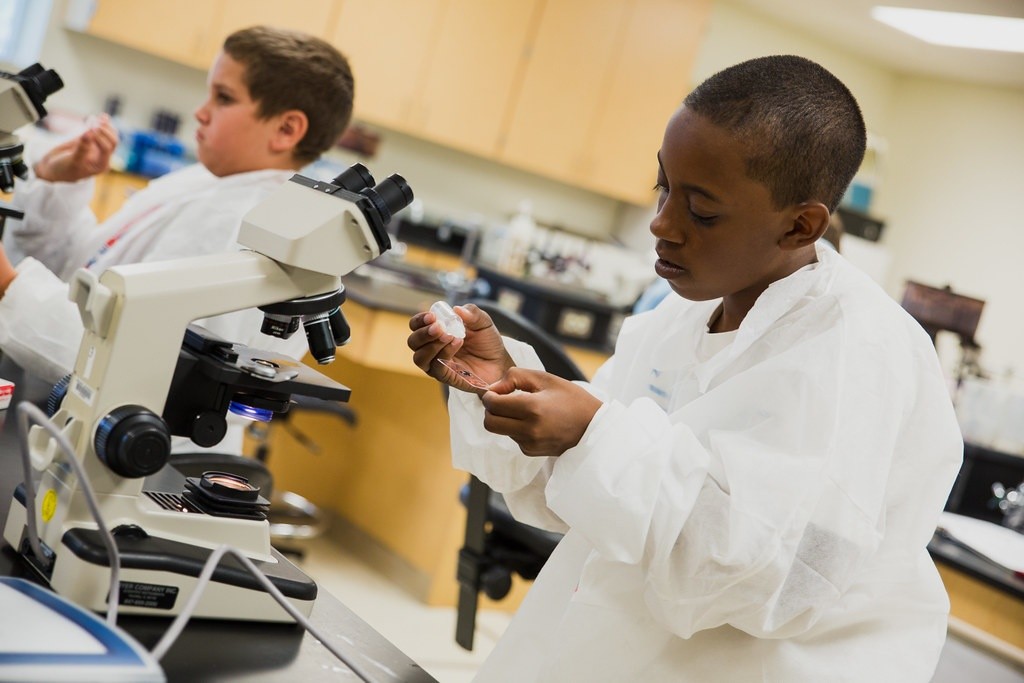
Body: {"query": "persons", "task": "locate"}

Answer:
[407,48,964,682]
[0,23,355,395]
